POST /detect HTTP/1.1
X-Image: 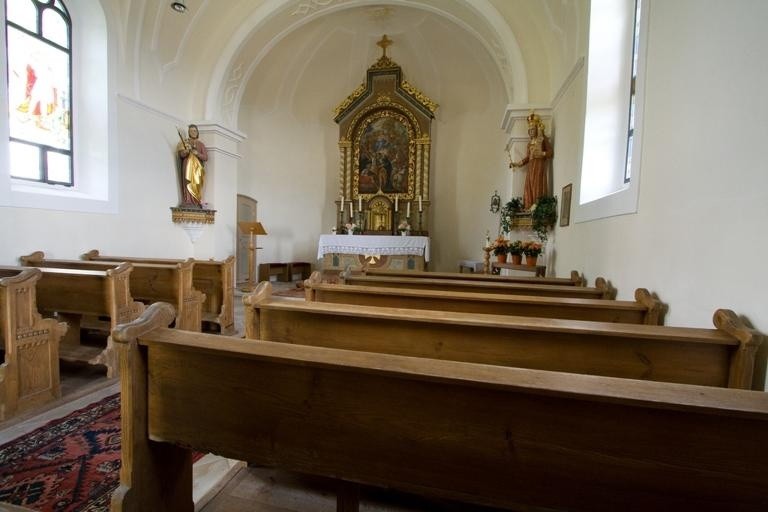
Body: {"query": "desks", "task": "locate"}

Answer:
[491,262,546,279]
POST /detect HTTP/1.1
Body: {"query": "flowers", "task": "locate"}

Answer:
[494,237,540,255]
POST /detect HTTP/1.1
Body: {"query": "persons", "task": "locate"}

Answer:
[173,122,209,212]
[372,203,388,232]
[510,112,554,210]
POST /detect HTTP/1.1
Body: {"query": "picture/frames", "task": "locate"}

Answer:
[561,183,571,228]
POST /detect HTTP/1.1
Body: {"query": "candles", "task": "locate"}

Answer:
[358,195,362,212]
[340,196,344,211]
[406,202,410,217]
[394,196,398,212]
[418,194,422,212]
[349,202,353,217]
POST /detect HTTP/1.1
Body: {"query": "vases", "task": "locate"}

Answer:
[496,254,537,265]
